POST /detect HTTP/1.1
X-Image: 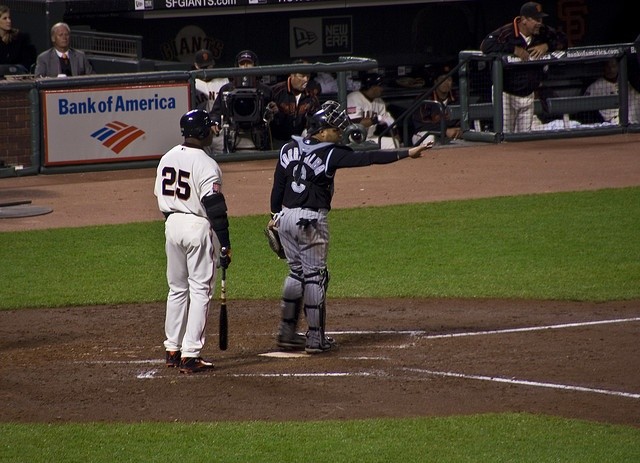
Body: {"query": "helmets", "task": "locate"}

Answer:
[306,100,351,139]
[180,108,217,140]
[360,72,383,90]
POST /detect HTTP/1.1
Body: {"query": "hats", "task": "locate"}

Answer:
[431,65,452,80]
[236,49,257,65]
[520,1,550,17]
[194,49,215,67]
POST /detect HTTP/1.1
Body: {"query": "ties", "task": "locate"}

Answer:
[62,53,68,65]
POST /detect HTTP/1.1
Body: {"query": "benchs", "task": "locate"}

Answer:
[545,95,624,129]
[449,98,546,131]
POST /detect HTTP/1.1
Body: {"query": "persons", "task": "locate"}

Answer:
[480,2,568,133]
[0,4,37,75]
[413,67,472,139]
[346,72,401,149]
[582,58,640,125]
[266,100,433,354]
[34,22,93,78]
[154,109,232,374]
[189,49,233,155]
[269,59,321,150]
[209,50,279,151]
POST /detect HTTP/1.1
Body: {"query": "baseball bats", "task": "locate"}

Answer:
[219,248,227,351]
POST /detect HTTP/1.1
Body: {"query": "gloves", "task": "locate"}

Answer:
[219,254,231,269]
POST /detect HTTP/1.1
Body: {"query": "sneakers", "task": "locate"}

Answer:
[276,333,306,348]
[179,357,214,372]
[305,336,335,353]
[165,351,180,366]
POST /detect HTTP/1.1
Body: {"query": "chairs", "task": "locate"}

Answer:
[320,80,435,143]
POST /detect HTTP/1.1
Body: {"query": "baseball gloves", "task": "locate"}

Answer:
[264,218,286,259]
